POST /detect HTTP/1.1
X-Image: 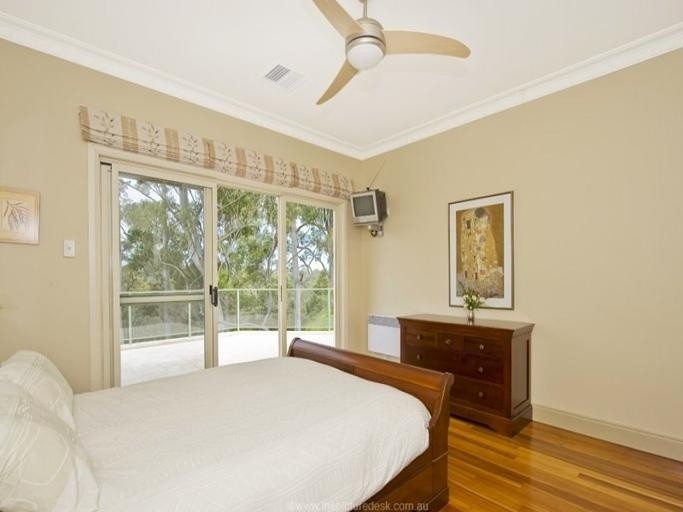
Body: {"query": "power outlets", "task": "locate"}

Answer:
[63,240,75,257]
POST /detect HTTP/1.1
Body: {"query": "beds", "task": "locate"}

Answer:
[0,338,456,512]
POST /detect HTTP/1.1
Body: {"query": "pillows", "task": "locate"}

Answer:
[0,349,77,434]
[0,375,101,512]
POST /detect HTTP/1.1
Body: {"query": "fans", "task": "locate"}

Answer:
[313,0,472,106]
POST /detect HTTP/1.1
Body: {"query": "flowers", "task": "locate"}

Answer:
[455,279,485,310]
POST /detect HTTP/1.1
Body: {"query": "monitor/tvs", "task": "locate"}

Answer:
[350,186,388,226]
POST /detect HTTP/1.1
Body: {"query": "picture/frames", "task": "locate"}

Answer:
[0,185,41,246]
[447,190,514,311]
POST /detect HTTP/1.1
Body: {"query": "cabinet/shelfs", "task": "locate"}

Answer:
[396,314,535,438]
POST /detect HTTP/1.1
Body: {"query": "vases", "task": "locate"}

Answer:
[466,310,474,322]
